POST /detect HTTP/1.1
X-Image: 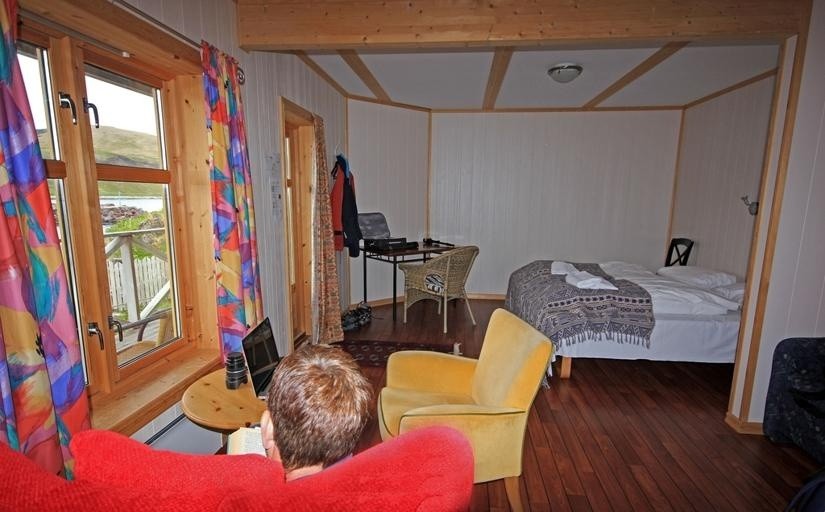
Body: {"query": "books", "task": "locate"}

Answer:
[226,422,269,461]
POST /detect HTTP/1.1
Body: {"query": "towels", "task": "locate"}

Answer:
[552,263,579,274]
[565,271,618,290]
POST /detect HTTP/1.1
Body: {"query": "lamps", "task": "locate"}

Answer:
[548,62,583,83]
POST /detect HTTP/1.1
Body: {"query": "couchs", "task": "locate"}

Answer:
[0,424,473,511]
[763,334,825,470]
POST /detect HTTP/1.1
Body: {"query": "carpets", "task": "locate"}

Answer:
[329,340,463,367]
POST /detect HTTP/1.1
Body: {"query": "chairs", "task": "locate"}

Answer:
[665,238,694,266]
[399,245,479,333]
[379,307,556,512]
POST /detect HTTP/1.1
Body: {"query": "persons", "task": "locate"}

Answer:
[260,342,375,481]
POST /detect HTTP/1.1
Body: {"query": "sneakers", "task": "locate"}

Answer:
[341,298,372,332]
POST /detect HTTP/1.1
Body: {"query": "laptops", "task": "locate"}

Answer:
[241,316,280,400]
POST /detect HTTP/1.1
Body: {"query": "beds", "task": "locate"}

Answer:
[504,258,746,389]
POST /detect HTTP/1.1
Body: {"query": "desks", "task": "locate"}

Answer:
[358,241,458,320]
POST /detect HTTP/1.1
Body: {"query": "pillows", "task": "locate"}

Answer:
[658,266,736,288]
[706,281,745,302]
